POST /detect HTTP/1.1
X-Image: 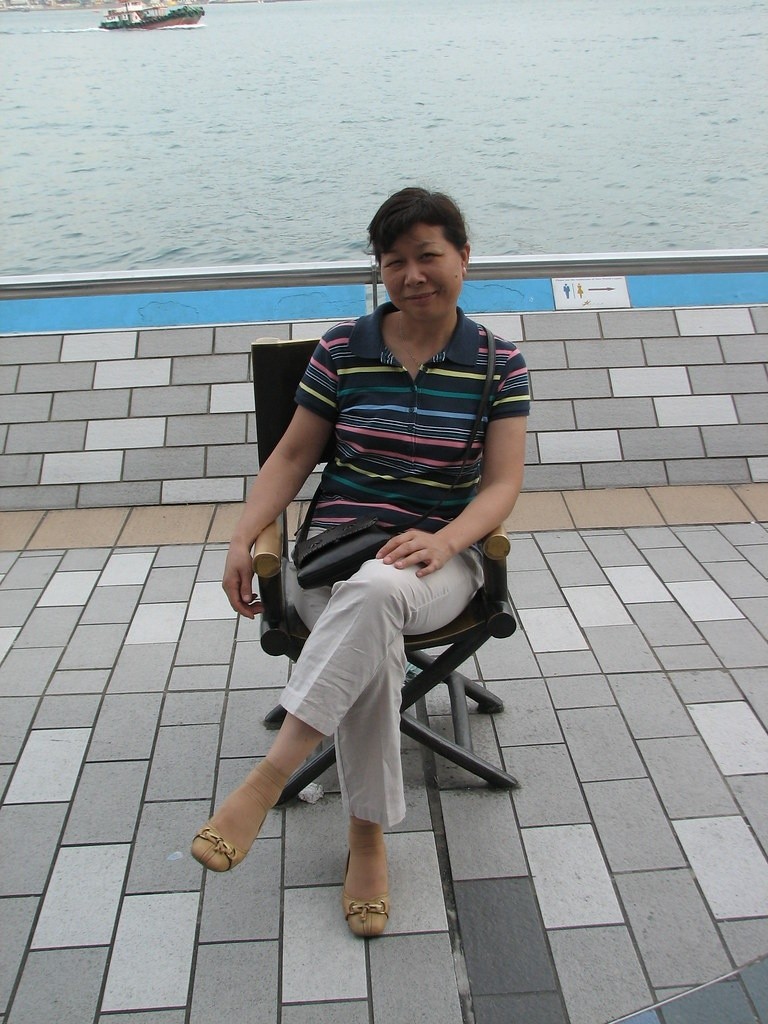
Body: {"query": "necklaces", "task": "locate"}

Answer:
[399,313,422,367]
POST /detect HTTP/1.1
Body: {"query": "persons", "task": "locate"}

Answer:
[190,187,531,938]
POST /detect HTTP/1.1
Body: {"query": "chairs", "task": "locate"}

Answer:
[250,338,519,806]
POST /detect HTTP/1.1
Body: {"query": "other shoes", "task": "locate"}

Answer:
[342,846,390,936]
[191,812,266,873]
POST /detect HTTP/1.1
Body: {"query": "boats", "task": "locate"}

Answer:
[97,0,206,30]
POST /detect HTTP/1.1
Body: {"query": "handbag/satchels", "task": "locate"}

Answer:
[296,515,394,589]
